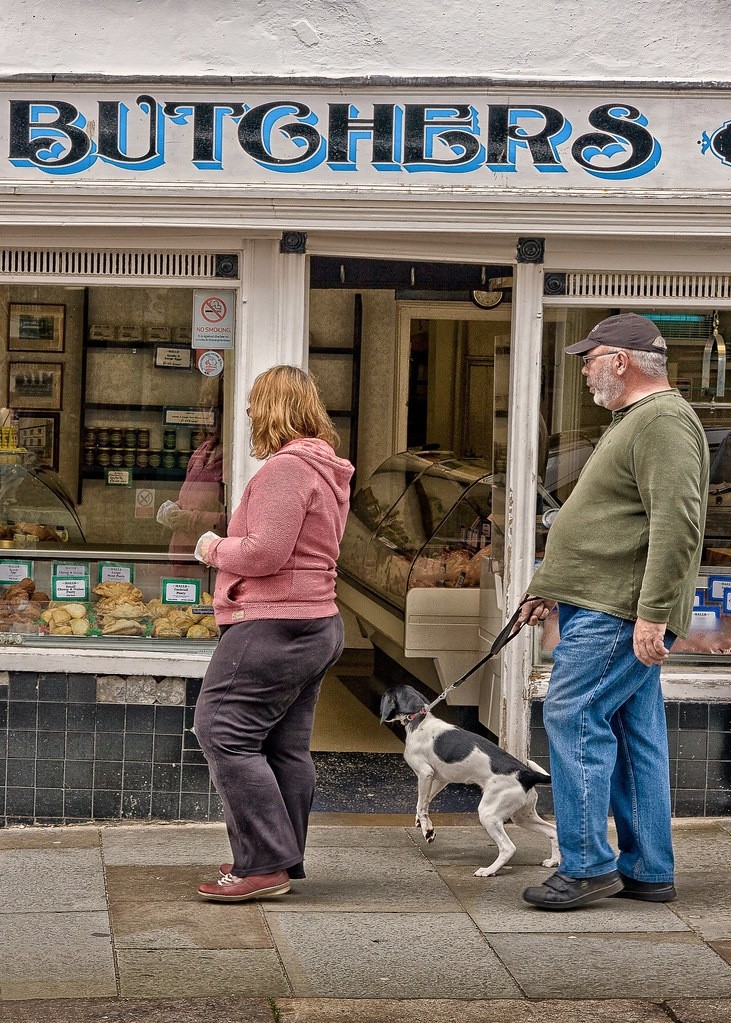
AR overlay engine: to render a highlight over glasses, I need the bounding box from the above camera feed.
[246,407,252,418]
[582,352,630,366]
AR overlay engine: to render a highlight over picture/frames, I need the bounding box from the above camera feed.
[11,413,61,472]
[7,303,66,352]
[8,361,64,410]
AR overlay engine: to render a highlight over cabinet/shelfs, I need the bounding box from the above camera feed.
[76,287,226,505]
[309,292,364,498]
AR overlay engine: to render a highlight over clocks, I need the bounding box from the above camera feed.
[471,291,504,310]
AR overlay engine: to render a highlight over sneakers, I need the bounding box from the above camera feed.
[219,863,235,876]
[197,867,291,902]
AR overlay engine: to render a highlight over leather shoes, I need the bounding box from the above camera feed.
[607,871,678,902]
[523,868,624,908]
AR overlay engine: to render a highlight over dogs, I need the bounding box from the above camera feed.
[377,686,562,878]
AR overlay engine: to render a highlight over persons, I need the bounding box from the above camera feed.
[168,373,226,596]
[513,313,710,909]
[0,446,83,542]
[194,365,355,901]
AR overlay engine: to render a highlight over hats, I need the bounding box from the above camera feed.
[563,312,667,355]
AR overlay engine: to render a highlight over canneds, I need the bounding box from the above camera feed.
[83,426,214,470]
